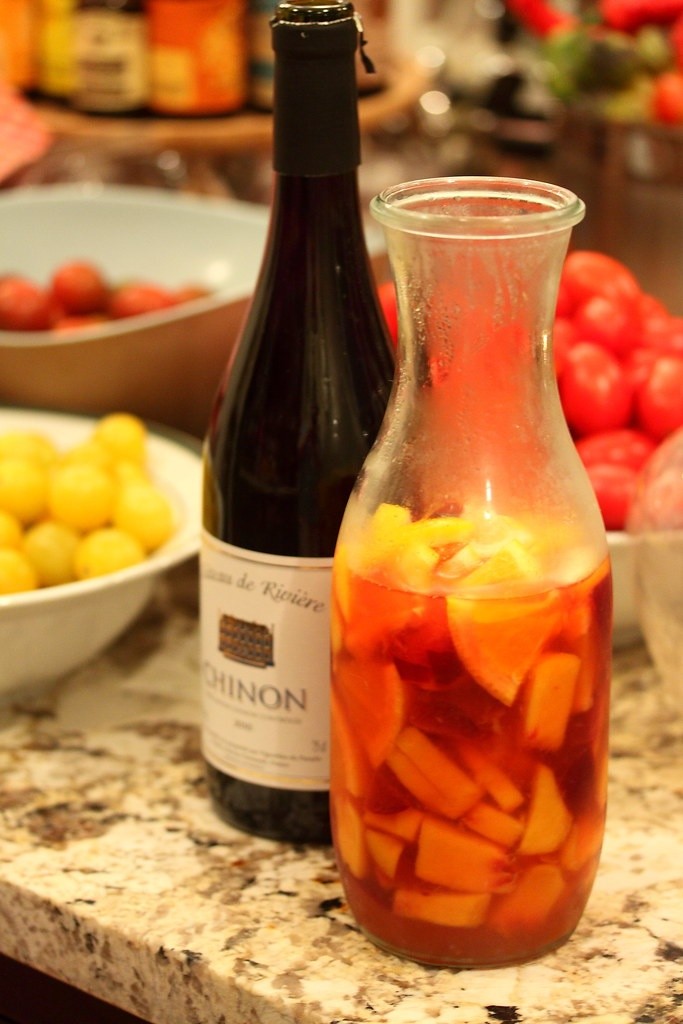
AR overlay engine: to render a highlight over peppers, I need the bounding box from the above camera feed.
[495,0,683,127]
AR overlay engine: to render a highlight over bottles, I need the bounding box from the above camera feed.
[324,172,618,970]
[199,0,422,846]
[27,0,386,120]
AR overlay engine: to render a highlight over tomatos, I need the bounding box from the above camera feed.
[0,413,176,596]
[377,252,683,531]
[1,261,210,336]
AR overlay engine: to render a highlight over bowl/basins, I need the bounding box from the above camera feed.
[1,178,291,435]
[605,526,683,717]
[1,403,226,700]
